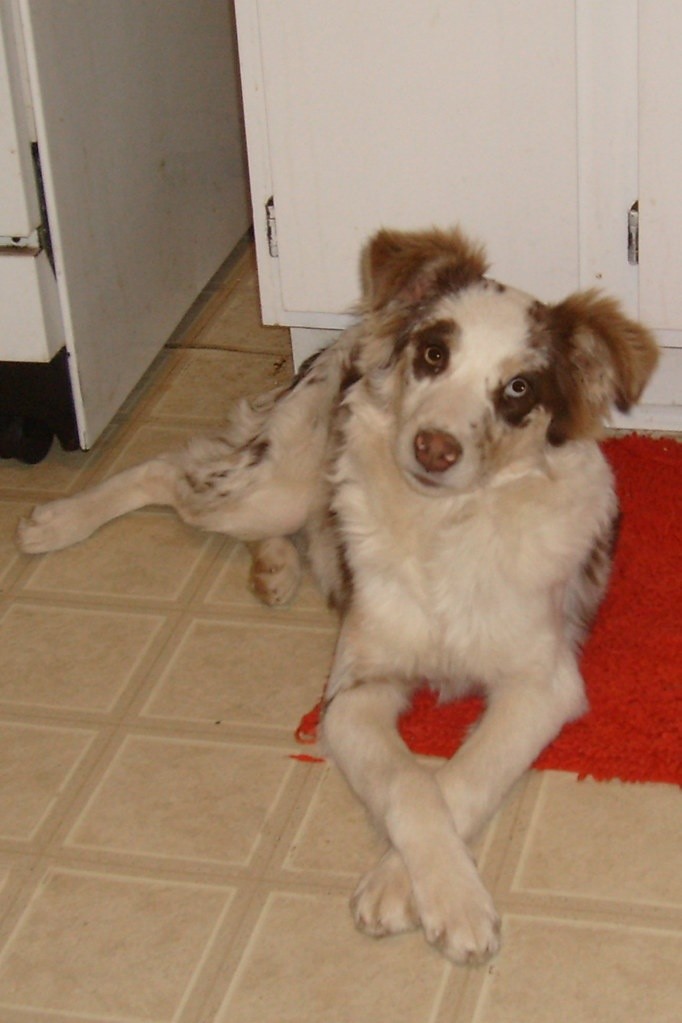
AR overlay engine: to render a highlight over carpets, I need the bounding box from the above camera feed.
[292,432,682,788]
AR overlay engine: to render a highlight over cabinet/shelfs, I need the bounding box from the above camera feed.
[0,0,248,452]
[235,0,682,432]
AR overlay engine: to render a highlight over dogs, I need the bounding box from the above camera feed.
[15,227,659,966]
